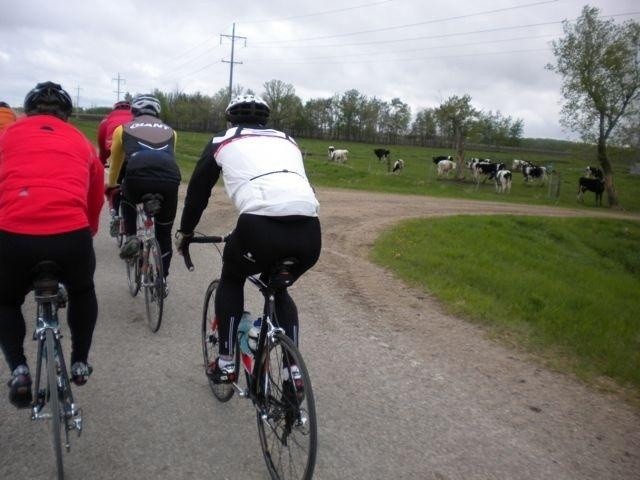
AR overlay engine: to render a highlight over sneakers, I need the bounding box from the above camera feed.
[155,277,170,298]
[119,235,140,259]
[109,216,120,237]
[278,379,307,404]
[71,361,93,385]
[8,373,33,408]
[204,358,238,383]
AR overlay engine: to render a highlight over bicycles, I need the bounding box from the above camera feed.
[102,162,141,268]
[14,256,88,479]
[182,231,319,480]
[111,191,172,337]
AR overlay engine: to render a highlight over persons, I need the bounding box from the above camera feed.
[0,80,106,409]
[0,100,17,132]
[105,93,183,296]
[177,92,324,402]
[97,99,134,237]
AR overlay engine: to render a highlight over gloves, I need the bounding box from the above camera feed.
[174,227,193,255]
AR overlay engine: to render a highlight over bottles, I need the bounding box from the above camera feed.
[248,317,265,352]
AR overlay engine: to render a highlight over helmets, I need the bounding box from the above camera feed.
[131,94,163,114]
[112,98,130,108]
[23,83,72,107]
[225,94,271,115]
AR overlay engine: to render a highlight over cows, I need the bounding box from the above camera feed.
[393,158,405,176]
[374,148,391,160]
[327,145,349,163]
[464,157,512,194]
[584,165,605,181]
[432,155,457,177]
[577,177,606,208]
[512,159,547,184]
